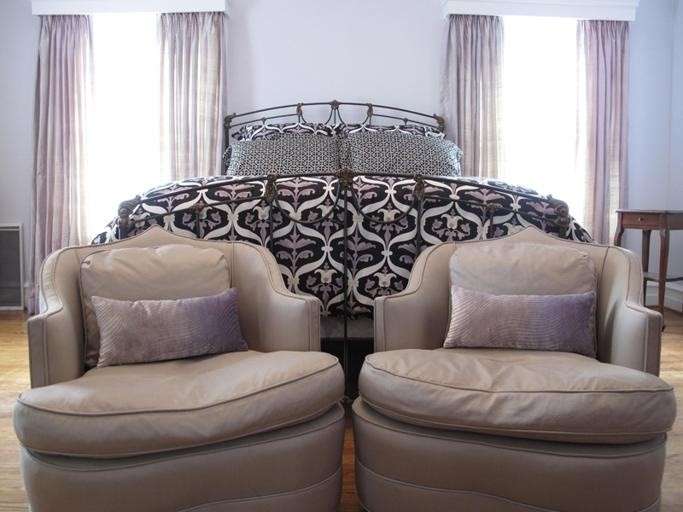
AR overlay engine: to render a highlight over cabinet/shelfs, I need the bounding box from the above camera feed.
[0,222,24,312]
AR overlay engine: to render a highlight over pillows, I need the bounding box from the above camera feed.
[231,122,339,142]
[77,243,232,368]
[89,285,248,370]
[222,135,340,176]
[444,239,598,356]
[335,123,446,139]
[441,282,600,356]
[341,131,464,177]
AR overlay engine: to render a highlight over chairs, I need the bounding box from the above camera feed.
[12,223,348,511]
[349,222,677,512]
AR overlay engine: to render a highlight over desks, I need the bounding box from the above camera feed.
[611,208,682,333]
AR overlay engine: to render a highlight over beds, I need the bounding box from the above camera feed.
[85,99,598,404]
